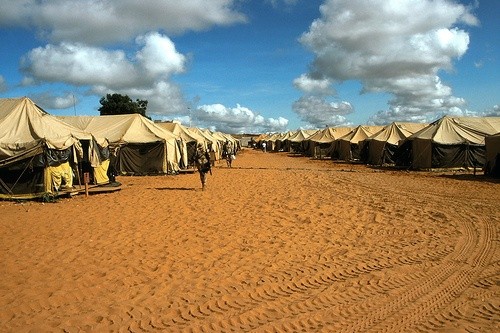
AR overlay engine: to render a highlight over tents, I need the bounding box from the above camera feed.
[0,95,499,199]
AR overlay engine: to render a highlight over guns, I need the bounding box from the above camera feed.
[205,149,212,175]
